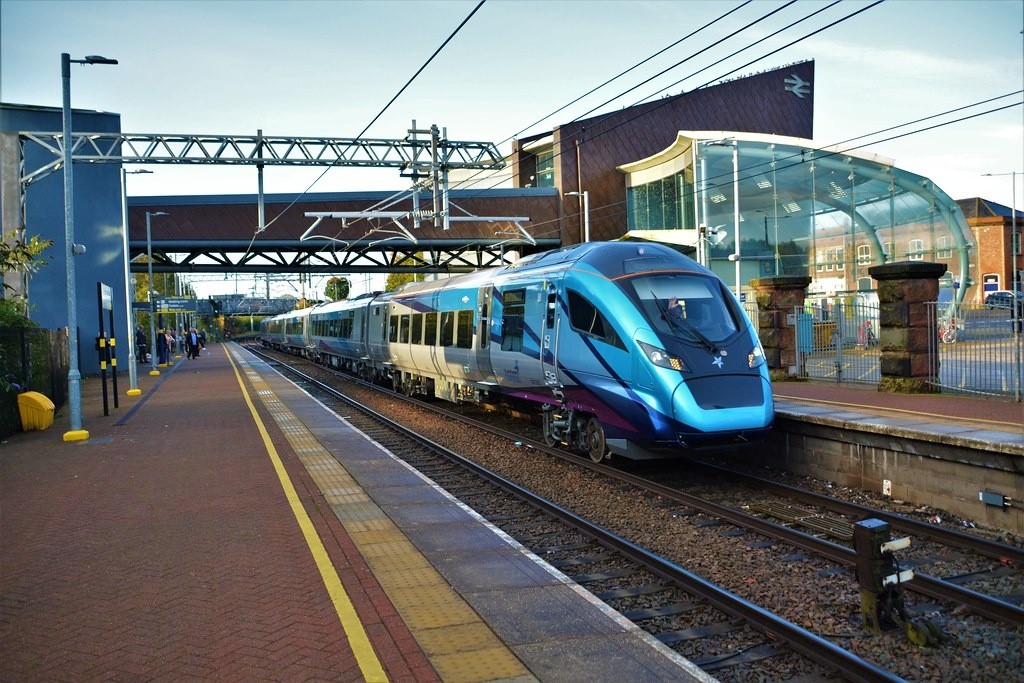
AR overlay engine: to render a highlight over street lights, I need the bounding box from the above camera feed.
[145,211,170,375]
[118,167,154,396]
[61,52,120,442]
[564,190,590,244]
[160,252,199,365]
[698,224,728,268]
[979,171,1024,403]
[707,139,741,304]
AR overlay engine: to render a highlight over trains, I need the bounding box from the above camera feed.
[260,240,773,464]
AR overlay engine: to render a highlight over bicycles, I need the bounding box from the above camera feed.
[864,316,876,349]
[937,316,959,344]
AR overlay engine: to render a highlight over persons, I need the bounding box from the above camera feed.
[666,296,682,326]
[136,325,150,363]
[157,327,206,363]
[99,331,111,378]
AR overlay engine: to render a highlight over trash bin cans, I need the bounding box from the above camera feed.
[17,391,56,431]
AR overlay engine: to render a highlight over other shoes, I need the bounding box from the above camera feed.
[187,356,190,360]
[193,358,196,359]
[144,361,151,363]
[140,362,145,363]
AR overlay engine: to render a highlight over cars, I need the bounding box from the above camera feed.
[983,290,1024,314]
